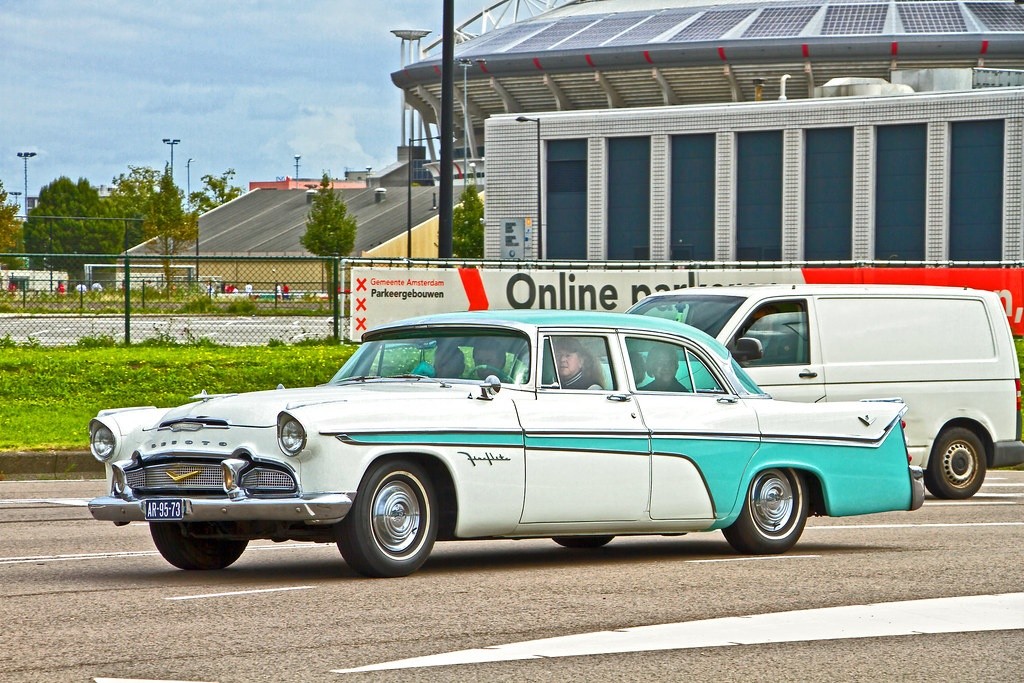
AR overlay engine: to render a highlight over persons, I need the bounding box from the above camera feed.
[553,337,605,390]
[741,305,798,365]
[76,281,87,292]
[276,282,282,293]
[628,350,646,390]
[473,339,506,380]
[433,344,465,378]
[91,281,102,292]
[8,280,18,291]
[243,282,253,292]
[118,278,239,293]
[281,281,289,294]
[637,342,690,393]
[57,282,66,293]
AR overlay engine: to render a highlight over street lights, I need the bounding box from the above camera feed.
[163,138,179,182]
[15,150,38,252]
[8,191,20,207]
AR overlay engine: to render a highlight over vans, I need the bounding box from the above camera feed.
[628,283,1021,499]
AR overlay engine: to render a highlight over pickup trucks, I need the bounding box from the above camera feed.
[83,305,924,573]
